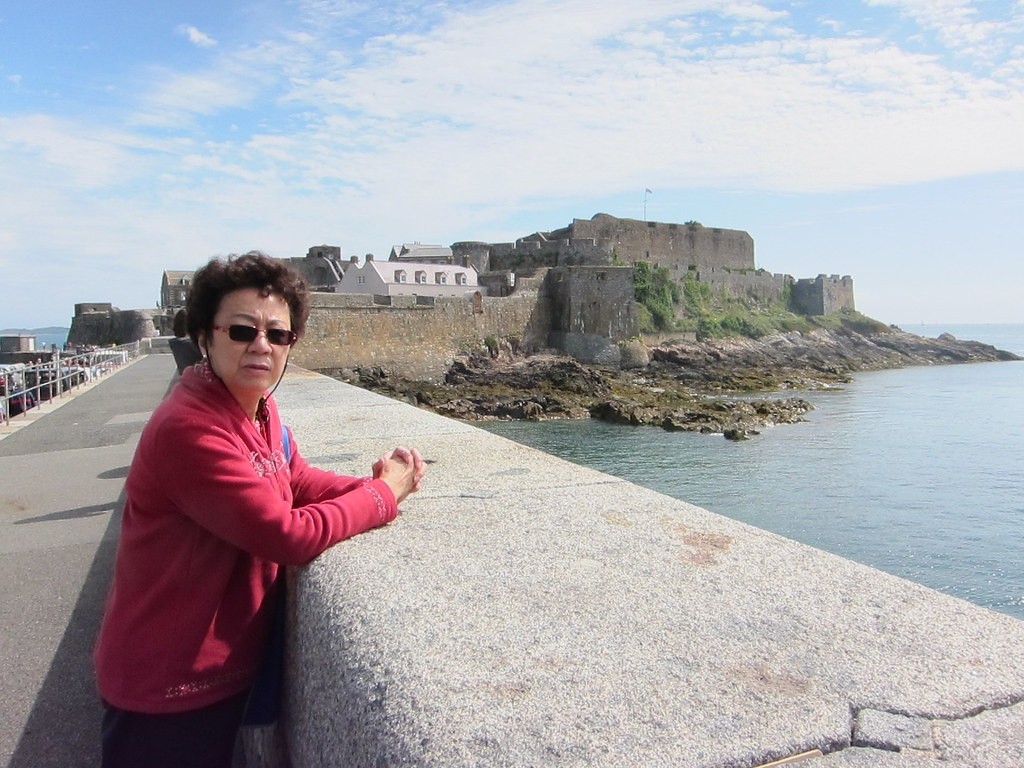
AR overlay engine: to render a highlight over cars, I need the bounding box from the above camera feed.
[0,367,35,419]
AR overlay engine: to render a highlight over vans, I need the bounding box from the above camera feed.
[22,367,89,401]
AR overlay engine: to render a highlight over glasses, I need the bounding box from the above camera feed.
[213,324,298,345]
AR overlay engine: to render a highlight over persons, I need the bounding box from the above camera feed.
[95,255,425,768]
